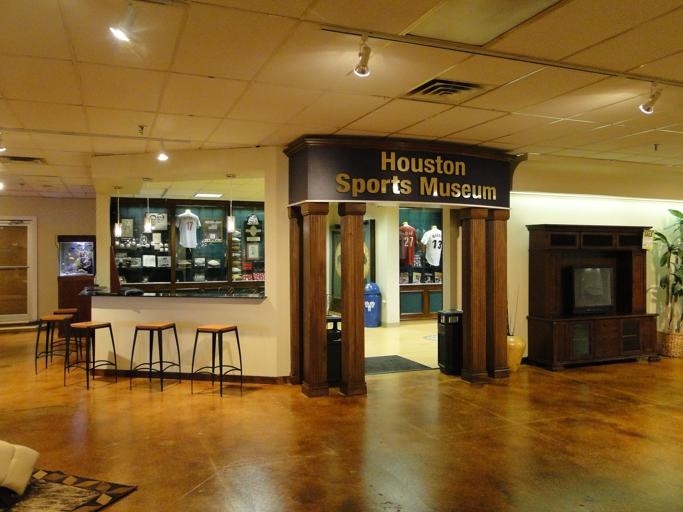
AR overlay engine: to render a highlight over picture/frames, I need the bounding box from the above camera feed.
[246,242,260,261]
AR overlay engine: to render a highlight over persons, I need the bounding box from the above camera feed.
[421,224,443,267]
[150,215,156,225]
[399,221,416,267]
[175,209,201,248]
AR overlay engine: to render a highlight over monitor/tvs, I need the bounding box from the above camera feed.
[562,265,616,316]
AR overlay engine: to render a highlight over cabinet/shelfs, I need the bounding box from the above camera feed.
[522,224,659,372]
[109,195,265,292]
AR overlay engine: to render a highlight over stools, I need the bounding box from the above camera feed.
[191,324,242,397]
[130,321,181,391]
[63,321,117,390]
[35,314,78,374]
[49,307,83,364]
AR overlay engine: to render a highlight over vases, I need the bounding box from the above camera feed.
[507,336,526,372]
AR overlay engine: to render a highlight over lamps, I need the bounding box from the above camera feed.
[354,43,370,75]
[639,91,661,113]
[108,3,138,41]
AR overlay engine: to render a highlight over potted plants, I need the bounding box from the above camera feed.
[652,208,683,357]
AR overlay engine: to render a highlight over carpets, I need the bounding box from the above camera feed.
[0,468,138,511]
[365,355,433,375]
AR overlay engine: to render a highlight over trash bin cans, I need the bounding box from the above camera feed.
[437,309,463,375]
[326,317,341,387]
[364,282,381,327]
